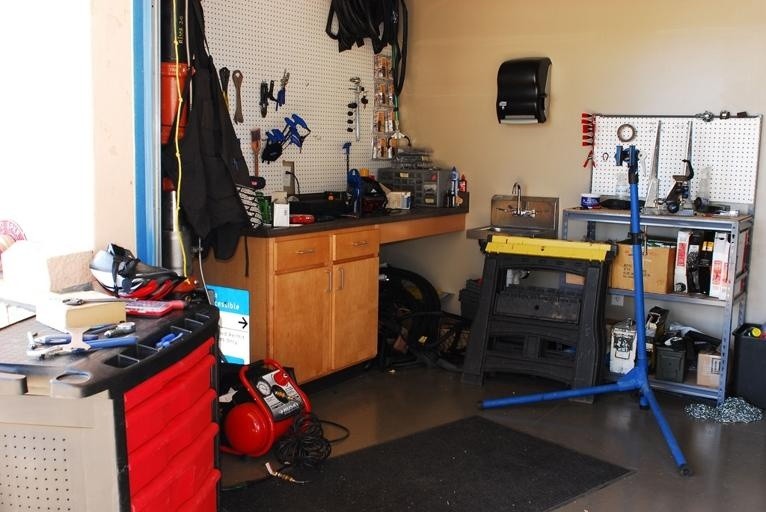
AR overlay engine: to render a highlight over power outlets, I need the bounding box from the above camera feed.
[283,168,291,187]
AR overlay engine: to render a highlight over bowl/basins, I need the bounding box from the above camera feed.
[580,194,601,208]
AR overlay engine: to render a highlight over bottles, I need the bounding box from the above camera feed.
[259,196,272,224]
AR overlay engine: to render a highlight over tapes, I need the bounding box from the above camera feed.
[617,124,634,142]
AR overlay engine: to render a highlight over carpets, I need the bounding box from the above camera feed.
[220,417,637,512]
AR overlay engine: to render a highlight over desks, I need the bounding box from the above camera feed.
[191,213,466,394]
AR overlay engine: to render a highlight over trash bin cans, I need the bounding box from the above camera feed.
[732,323,766,410]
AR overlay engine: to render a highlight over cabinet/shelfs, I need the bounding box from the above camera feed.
[561,206,754,409]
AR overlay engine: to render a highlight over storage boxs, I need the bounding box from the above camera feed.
[611,240,677,295]
[566,261,611,287]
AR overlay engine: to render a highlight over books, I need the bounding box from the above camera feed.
[35,291,126,334]
